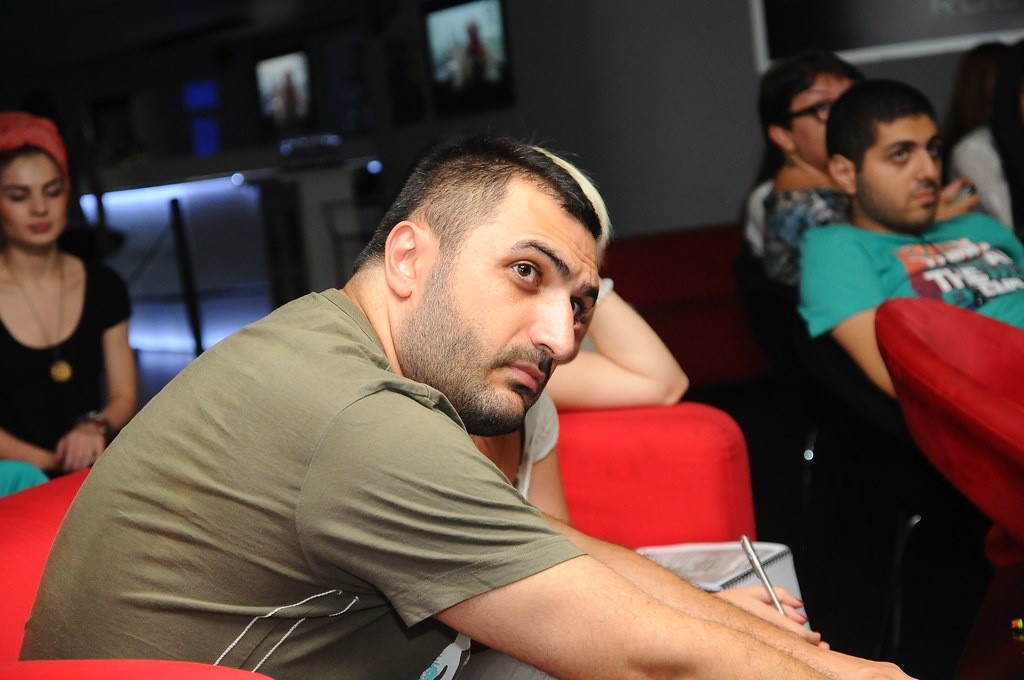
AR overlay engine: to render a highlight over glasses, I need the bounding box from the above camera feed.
[789,98,834,123]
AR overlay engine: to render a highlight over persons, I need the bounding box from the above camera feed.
[0,110,141,498]
[19,119,921,680]
[741,38,1024,400]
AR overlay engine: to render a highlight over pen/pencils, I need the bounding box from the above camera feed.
[739,535,788,620]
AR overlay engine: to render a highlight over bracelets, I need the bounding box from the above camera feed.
[595,277,613,303]
[84,410,116,444]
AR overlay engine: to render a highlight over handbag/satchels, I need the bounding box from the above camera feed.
[452,534,812,680]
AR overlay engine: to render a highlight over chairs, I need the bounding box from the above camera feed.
[873,296,1024,680]
[0,400,754,680]
[600,222,772,392]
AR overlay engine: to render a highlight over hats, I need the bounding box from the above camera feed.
[0,112,71,197]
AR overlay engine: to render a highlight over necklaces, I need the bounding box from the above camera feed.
[3,257,71,380]
